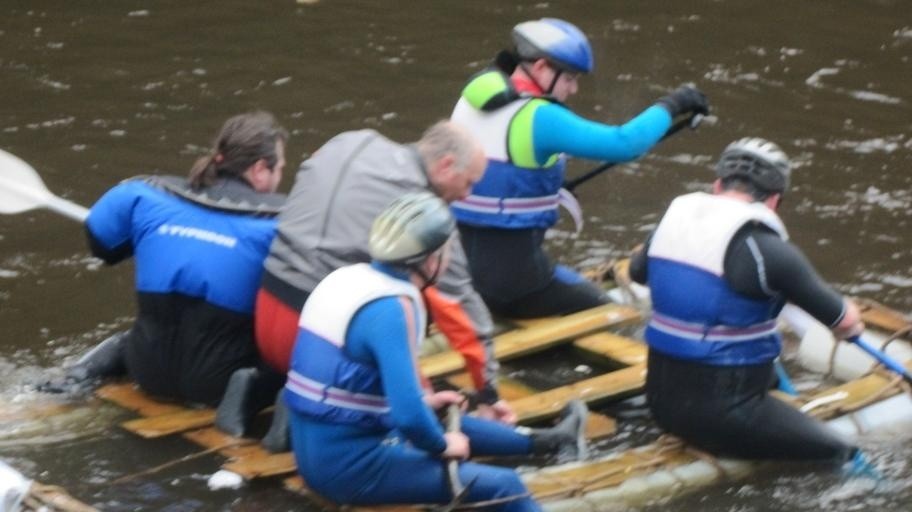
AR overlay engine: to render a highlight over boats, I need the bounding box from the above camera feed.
[33,257,911,512]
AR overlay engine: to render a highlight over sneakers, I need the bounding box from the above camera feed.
[544,397,587,462]
[261,386,291,452]
[215,365,261,437]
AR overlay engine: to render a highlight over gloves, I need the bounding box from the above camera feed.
[658,80,710,117]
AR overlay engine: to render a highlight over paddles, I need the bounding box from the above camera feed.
[431,377,478,512]
[0,147,89,222]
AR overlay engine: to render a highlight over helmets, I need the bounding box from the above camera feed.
[368,189,456,266]
[511,15,594,75]
[715,136,791,199]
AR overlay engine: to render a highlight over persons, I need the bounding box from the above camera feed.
[445,17,709,322]
[630,134,876,477]
[33,109,296,403]
[211,117,522,454]
[283,190,588,511]
